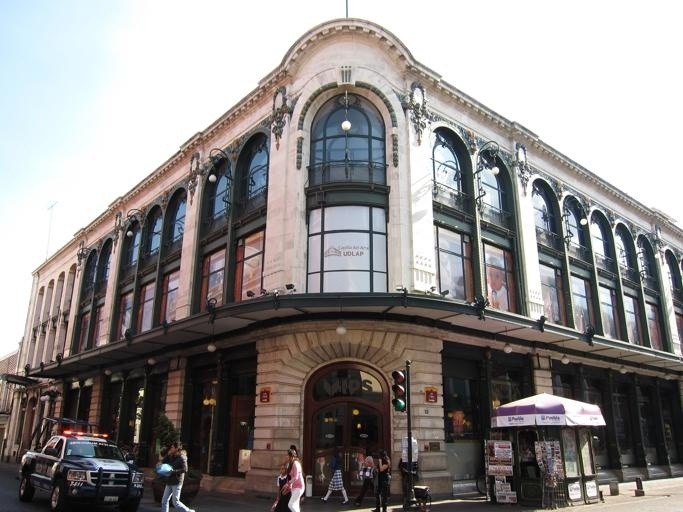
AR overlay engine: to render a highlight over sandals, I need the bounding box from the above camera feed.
[320,497,327,502]
[341,500,349,505]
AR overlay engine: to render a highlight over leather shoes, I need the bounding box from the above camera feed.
[354,503,361,506]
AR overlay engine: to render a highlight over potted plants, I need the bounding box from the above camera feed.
[151,411,204,507]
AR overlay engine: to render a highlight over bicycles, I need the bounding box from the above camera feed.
[476,468,487,496]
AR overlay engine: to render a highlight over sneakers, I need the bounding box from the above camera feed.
[371,508,387,512]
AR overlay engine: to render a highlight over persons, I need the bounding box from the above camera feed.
[319,446,350,505]
[371,448,392,512]
[355,447,364,481]
[285,444,298,469]
[353,455,378,507]
[270,464,292,512]
[280,448,305,512]
[317,450,326,479]
[154,439,195,512]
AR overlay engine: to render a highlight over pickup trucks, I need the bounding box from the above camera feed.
[19,430,144,512]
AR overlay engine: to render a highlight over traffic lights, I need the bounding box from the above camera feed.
[392,369,406,412]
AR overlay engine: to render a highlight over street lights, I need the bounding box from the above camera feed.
[203,398,217,473]
[40,381,63,441]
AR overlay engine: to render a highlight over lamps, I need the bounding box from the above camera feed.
[474,140,500,220]
[126,208,149,256]
[564,193,588,251]
[341,89,352,179]
[209,148,233,208]
